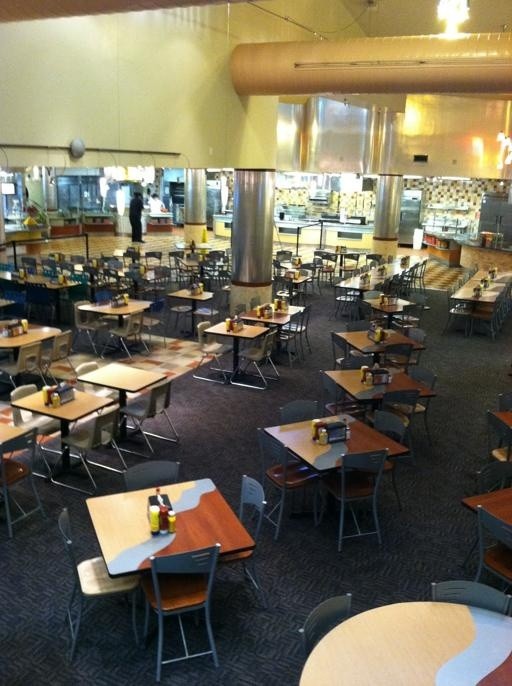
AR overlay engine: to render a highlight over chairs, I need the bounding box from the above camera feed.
[302,593,351,661]
[323,448,382,555]
[219,474,268,611]
[430,580,511,616]
[462,393,510,593]
[270,244,426,320]
[58,508,142,660]
[279,400,318,461]
[196,302,313,392]
[1,234,235,360]
[373,411,403,512]
[442,264,510,342]
[144,543,220,682]
[258,428,319,541]
[1,330,184,538]
[316,292,437,444]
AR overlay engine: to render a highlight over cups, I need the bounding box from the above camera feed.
[279,212,284,220]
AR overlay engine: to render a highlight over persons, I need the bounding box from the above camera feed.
[148,193,166,214]
[129,192,147,244]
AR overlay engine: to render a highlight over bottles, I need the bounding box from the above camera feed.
[473,267,498,297]
[360,365,392,384]
[311,416,351,443]
[192,283,204,295]
[44,386,61,406]
[287,257,302,280]
[111,293,130,308]
[226,298,289,333]
[370,292,388,343]
[4,317,28,337]
[359,256,409,284]
[149,505,176,535]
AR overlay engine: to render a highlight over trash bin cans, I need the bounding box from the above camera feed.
[479,231,505,250]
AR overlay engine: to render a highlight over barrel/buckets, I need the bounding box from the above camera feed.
[493,232,503,249]
[482,232,494,248]
